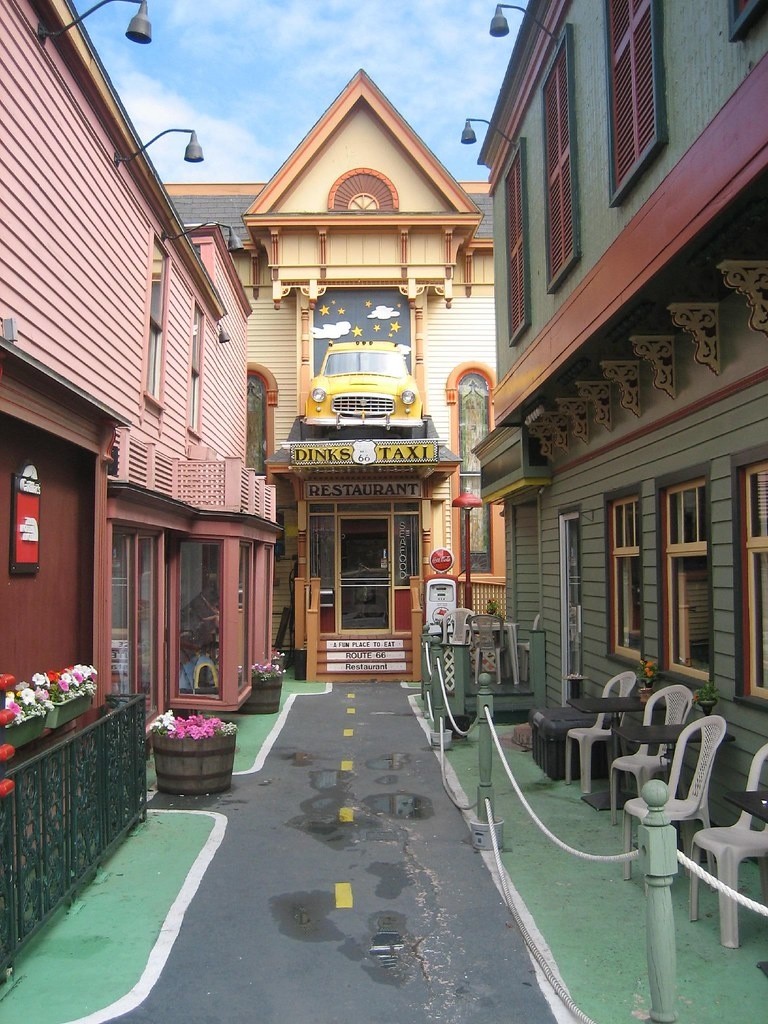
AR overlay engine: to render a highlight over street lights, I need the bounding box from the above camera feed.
[449,487,483,611]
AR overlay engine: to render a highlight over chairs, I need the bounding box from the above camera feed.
[623,715,726,892]
[442,607,476,646]
[517,614,541,681]
[468,614,508,685]
[689,743,768,948]
[566,671,636,793]
[611,684,695,824]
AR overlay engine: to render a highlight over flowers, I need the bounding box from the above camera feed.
[149,709,239,740]
[691,679,720,705]
[252,651,287,682]
[637,659,661,684]
[4,663,98,725]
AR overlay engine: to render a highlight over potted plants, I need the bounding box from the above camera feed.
[434,619,452,633]
[486,598,498,614]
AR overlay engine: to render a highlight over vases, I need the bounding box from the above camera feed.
[46,694,92,729]
[232,677,283,714]
[151,735,237,795]
[640,678,654,702]
[698,700,717,716]
[5,712,44,749]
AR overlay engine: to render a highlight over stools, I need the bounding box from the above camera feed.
[528,706,617,781]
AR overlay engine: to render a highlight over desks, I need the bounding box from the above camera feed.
[429,622,519,685]
[721,789,768,825]
[565,696,667,810]
[611,724,735,854]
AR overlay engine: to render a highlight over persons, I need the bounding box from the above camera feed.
[186,573,220,656]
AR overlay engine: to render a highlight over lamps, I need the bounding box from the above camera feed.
[39,0,152,44]
[161,221,244,252]
[217,323,231,343]
[490,5,559,46]
[114,129,204,168]
[461,119,521,149]
